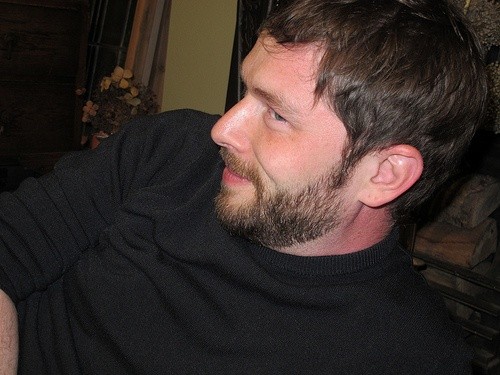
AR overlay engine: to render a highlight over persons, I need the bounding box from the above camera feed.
[0,0,489,375]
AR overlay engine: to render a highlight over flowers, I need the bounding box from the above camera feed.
[82,66,158,146]
